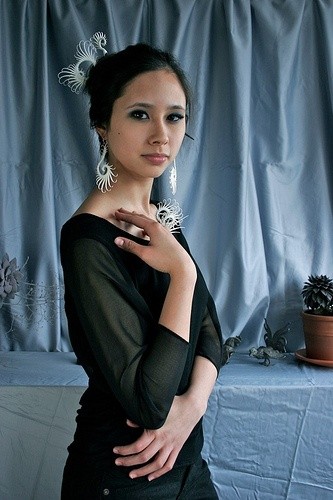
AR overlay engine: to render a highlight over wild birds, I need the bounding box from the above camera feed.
[223,336,288,367]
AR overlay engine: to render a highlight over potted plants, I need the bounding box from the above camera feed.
[301,273,333,360]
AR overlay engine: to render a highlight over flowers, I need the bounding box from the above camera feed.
[0,252,24,303]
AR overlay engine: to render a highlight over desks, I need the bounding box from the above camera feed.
[0,351,333,500]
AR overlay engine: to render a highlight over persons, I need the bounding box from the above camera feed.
[57,39,228,500]
